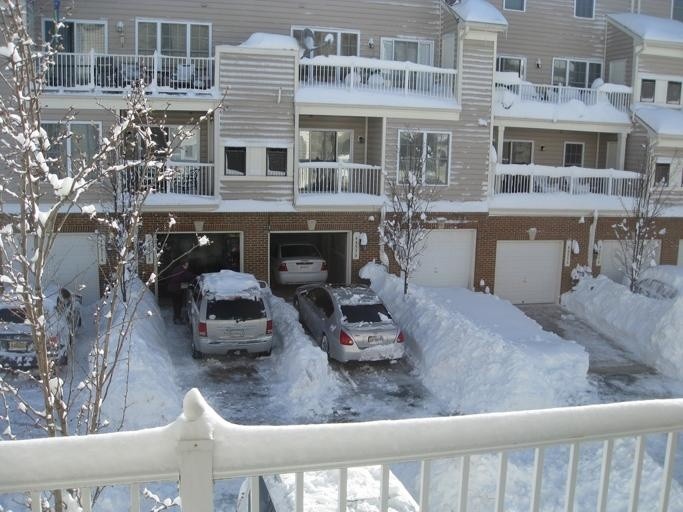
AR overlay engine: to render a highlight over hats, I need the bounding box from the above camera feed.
[182,270,273,358]
[272,243,328,285]
[293,282,403,362]
[631,264,683,302]
[1,285,83,373]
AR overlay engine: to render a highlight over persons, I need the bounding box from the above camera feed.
[167,259,196,325]
